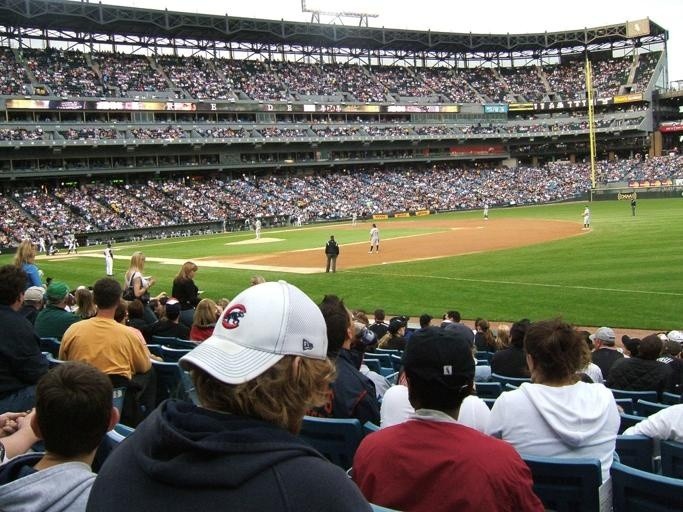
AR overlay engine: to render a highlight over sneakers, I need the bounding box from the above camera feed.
[324,271,336,273]
[368,251,379,254]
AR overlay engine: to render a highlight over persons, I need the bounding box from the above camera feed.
[315,296,682,511]
[253,217,262,240]
[0,47,372,512]
[324,236,340,273]
[250,56,682,229]
[367,224,380,254]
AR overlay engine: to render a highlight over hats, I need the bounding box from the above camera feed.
[402,326,475,381]
[351,323,377,345]
[23,281,69,301]
[388,317,407,333]
[589,327,616,344]
[657,330,683,342]
[177,278,330,385]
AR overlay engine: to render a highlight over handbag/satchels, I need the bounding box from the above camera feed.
[123,269,150,302]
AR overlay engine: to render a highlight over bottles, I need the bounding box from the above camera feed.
[354,322,377,345]
